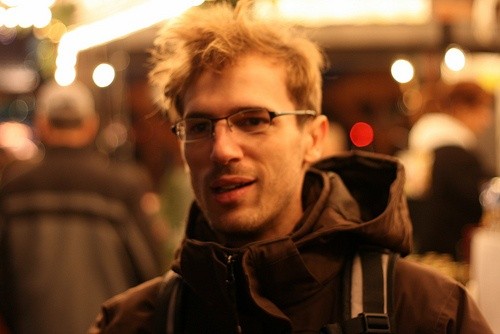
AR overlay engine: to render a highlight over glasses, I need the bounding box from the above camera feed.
[172,108,316,144]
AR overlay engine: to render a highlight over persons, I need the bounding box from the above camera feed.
[89,1,496,334]
[1,47,192,255]
[400,76,499,289]
